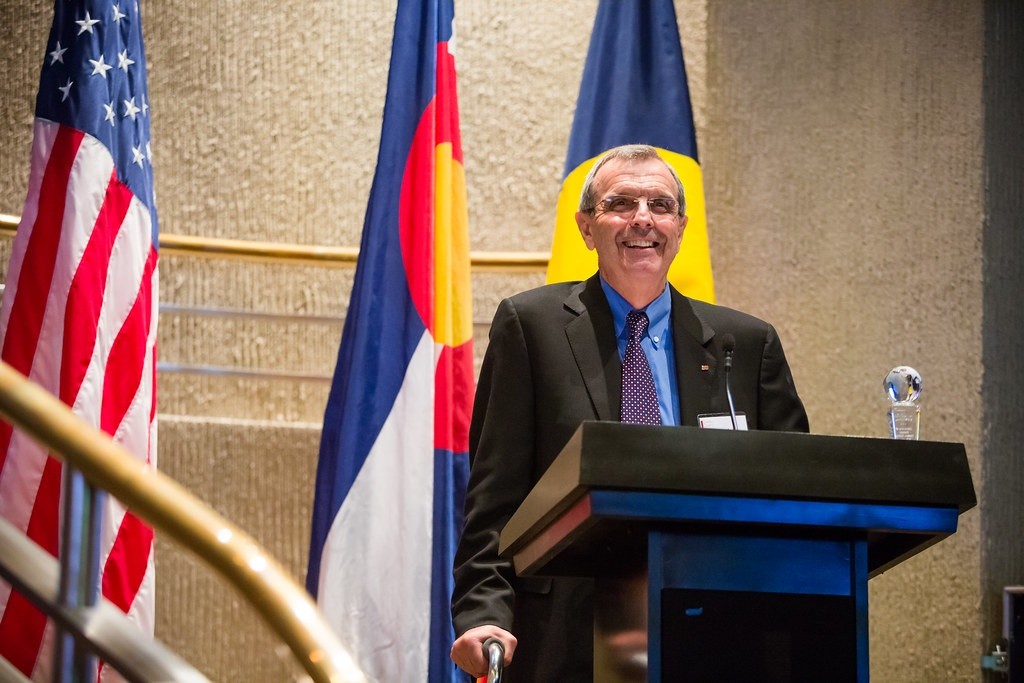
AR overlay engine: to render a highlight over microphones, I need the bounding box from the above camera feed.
[722,333,738,430]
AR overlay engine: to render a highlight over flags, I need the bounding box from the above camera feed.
[545,1,720,307]
[0,0,156,683]
[305,0,473,683]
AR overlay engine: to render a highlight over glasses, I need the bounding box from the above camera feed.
[588,195,681,216]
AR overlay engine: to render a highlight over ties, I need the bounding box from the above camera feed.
[619,310,661,426]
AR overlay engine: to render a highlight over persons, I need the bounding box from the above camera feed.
[449,144,810,675]
